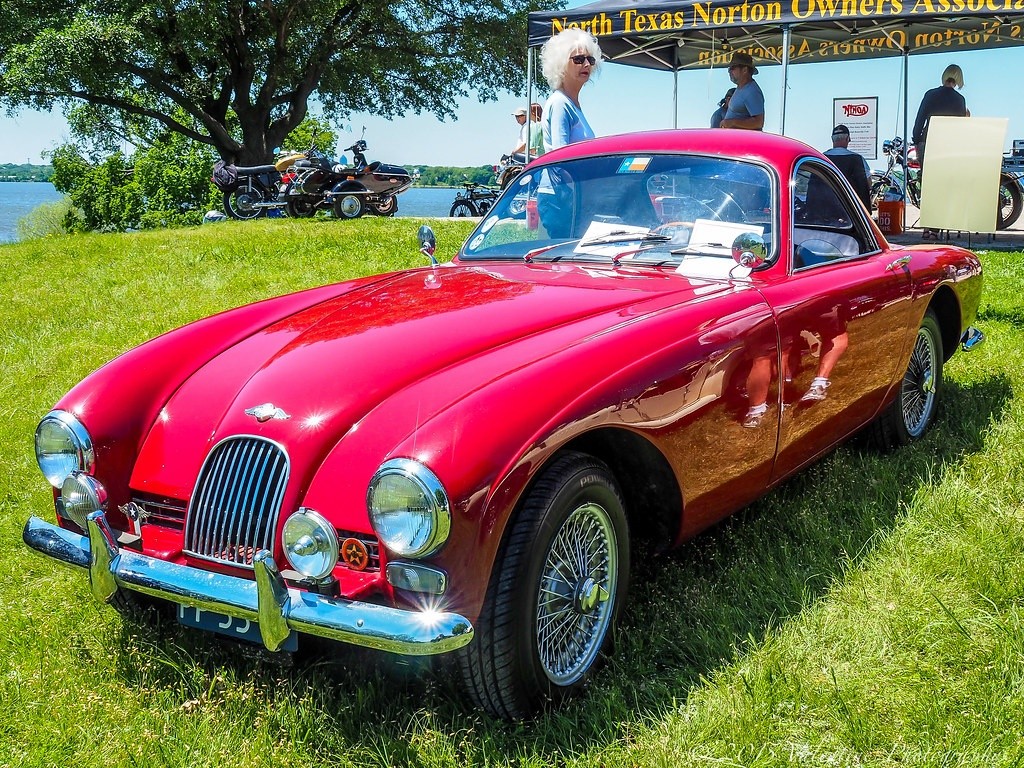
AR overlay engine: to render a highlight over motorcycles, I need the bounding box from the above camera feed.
[449,152,540,218]
[867,136,1024,231]
[209,124,421,218]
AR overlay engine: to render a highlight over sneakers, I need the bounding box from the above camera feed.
[800,381,832,402]
[743,405,770,428]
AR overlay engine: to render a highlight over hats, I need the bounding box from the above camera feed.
[729,53,759,75]
[511,109,527,115]
[833,125,851,142]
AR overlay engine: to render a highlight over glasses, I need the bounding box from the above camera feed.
[569,55,596,65]
[730,66,744,70]
[516,115,526,119]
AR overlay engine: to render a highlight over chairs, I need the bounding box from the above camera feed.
[805,154,872,226]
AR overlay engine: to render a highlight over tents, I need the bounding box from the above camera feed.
[528,0,1024,273]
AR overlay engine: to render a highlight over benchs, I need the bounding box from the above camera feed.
[762,227,860,260]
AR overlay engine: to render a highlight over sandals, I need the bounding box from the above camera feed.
[923,231,938,241]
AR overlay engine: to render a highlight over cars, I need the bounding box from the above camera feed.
[20,128,990,724]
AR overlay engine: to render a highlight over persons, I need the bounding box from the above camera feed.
[536,27,598,240]
[510,102,542,195]
[710,88,735,128]
[743,316,849,427]
[823,125,872,191]
[719,52,765,130]
[912,64,966,240]
